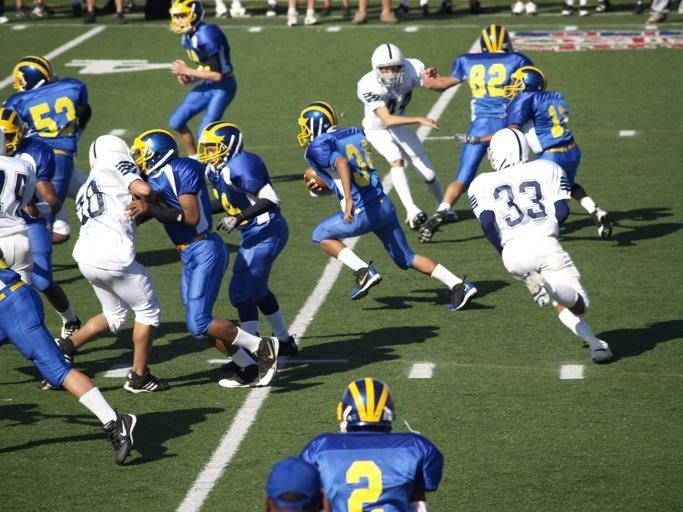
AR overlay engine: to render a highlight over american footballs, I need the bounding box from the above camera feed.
[304,168,333,194]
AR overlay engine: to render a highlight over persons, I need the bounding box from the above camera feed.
[296,373,446,512]
[461,126,618,367]
[126,129,279,388]
[167,0,238,163]
[451,62,615,242]
[0,55,92,339]
[262,455,326,512]
[1,1,683,28]
[0,247,137,465]
[40,135,171,394]
[198,121,298,363]
[298,98,479,312]
[415,22,535,244]
[354,42,461,231]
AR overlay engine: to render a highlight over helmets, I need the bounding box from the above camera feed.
[371,43,403,88]
[169,0,205,34]
[296,101,336,149]
[0,109,22,156]
[502,65,546,99]
[486,127,528,172]
[337,377,396,431]
[12,56,57,93]
[479,23,511,54]
[88,121,243,169]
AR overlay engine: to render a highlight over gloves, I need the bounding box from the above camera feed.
[454,133,480,145]
[216,213,243,233]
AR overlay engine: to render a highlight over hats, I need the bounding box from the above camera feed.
[266,457,321,509]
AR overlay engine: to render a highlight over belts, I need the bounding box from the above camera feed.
[0,280,25,301]
[544,140,576,153]
[175,228,213,251]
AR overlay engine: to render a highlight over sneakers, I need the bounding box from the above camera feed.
[406,205,457,243]
[590,338,613,362]
[349,260,382,301]
[123,369,168,394]
[39,338,73,390]
[103,414,137,464]
[217,335,297,390]
[447,275,478,312]
[595,210,611,239]
[61,315,80,339]
[522,271,552,307]
